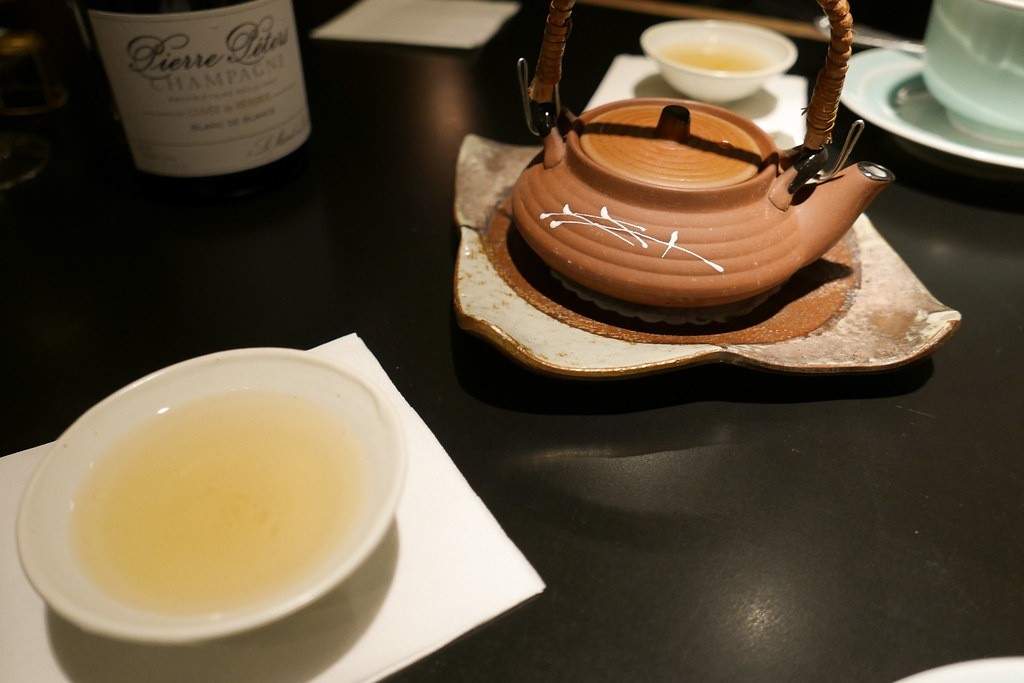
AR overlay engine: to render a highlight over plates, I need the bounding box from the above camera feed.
[839,44,1024,171]
[582,52,810,151]
[452,132,962,379]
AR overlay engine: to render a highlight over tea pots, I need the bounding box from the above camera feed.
[510,0,897,309]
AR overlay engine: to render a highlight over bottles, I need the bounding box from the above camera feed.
[75,0,324,221]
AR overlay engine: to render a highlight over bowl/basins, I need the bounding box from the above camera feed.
[16,345,401,645]
[921,1,1024,150]
[638,18,799,107]
